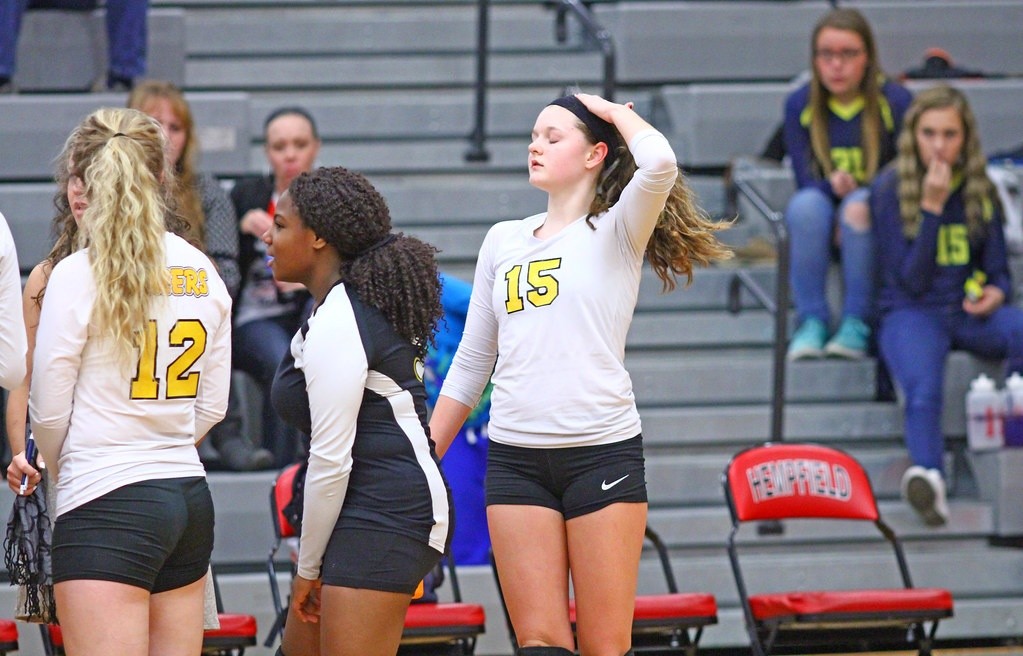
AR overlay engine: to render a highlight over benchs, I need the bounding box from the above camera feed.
[584,0,1023,493]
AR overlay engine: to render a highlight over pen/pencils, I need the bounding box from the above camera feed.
[19,433,38,496]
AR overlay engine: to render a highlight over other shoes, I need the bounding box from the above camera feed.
[900,464,951,526]
[785,315,827,362]
[823,316,873,361]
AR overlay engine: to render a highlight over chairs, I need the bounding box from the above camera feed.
[268,458,485,656]
[720,439,955,656]
[487,522,719,656]
[0,0,494,656]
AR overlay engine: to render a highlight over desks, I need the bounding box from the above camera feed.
[15,431,257,656]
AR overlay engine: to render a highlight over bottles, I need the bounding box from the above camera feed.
[1001,372,1023,450]
[966,372,1006,451]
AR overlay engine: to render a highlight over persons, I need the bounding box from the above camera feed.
[432,91,741,656]
[787,10,1023,527]
[0,0,499,656]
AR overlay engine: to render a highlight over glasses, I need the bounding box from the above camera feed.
[816,47,868,61]
